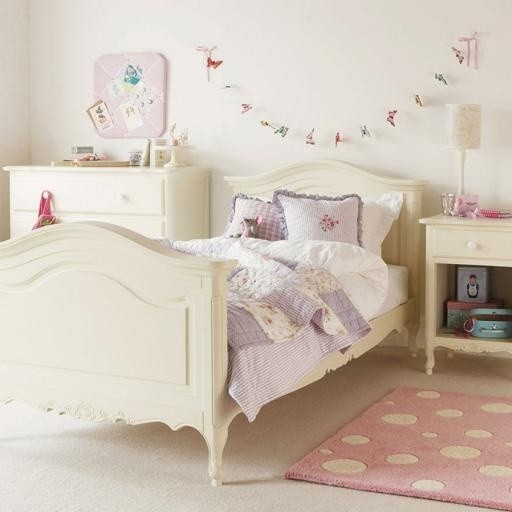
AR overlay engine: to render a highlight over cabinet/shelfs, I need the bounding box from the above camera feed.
[1,163,212,245]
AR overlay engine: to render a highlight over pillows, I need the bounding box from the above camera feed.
[272,188,364,247]
[224,192,286,243]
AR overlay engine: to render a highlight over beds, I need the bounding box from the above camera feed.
[0,156,432,485]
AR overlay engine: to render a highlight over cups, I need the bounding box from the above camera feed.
[438,192,456,216]
[128,149,144,166]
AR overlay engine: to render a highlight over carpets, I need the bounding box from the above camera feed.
[287,385,511,511]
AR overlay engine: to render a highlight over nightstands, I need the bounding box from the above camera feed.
[417,211,512,376]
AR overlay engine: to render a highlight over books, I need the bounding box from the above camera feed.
[481,210,512,218]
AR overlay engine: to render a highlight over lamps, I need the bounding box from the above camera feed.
[446,103,483,217]
